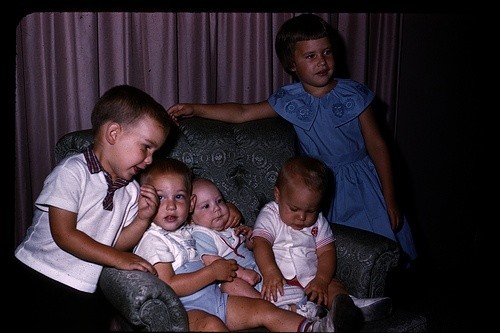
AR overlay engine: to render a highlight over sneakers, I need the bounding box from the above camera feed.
[312,293,364,333]
[296,301,328,321]
[351,297,394,322]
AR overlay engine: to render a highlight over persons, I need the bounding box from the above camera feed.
[12,84,172,331]
[166,14,418,271]
[131,156,366,331]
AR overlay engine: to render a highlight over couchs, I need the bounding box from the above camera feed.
[56,116,428,333]
